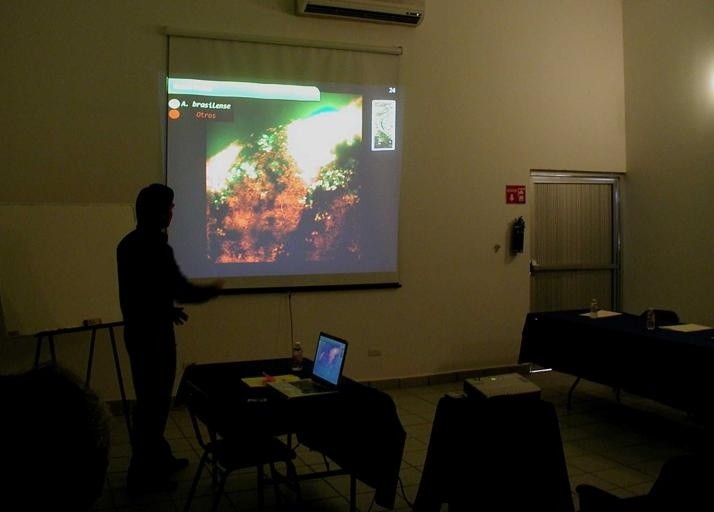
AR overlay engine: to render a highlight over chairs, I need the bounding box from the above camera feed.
[183,360,298,510]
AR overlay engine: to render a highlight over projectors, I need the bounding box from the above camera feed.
[463,372,541,405]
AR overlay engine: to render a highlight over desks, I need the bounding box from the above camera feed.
[519,312,710,429]
[180,357,406,509]
[416,392,575,510]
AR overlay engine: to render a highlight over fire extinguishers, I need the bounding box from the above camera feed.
[511,216,525,252]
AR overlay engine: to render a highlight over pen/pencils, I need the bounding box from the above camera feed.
[248,399,267,402]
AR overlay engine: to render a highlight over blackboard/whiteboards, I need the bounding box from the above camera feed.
[0,202,137,339]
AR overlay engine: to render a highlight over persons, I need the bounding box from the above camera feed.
[1,363,111,512]
[117,184,225,494]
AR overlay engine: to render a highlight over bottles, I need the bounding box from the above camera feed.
[590,299,598,320]
[645,308,656,331]
[290,341,304,372]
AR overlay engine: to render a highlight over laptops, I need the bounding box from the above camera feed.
[266,331,348,399]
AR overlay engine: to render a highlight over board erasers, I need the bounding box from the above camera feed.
[84,318,102,326]
